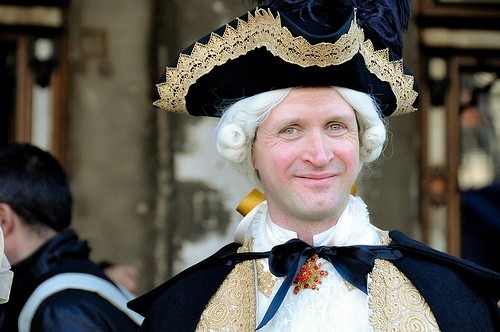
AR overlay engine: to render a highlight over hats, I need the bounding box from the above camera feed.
[151,0,421,119]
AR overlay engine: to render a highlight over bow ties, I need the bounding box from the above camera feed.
[222,239,403,331]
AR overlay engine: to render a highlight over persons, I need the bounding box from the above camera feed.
[123,0,500,331]
[0,142,147,332]
